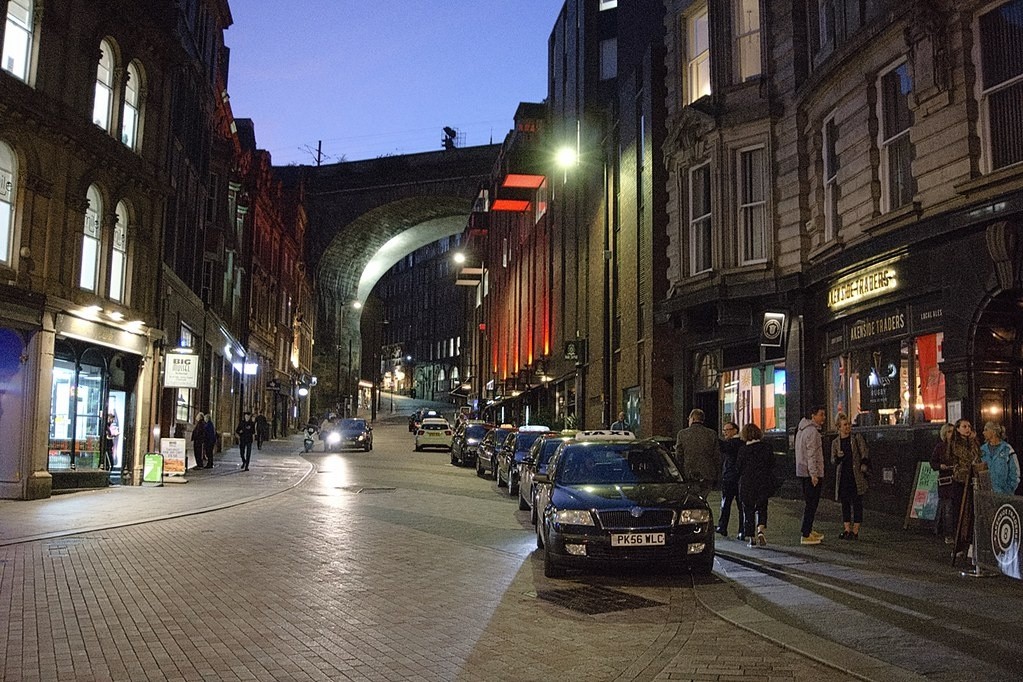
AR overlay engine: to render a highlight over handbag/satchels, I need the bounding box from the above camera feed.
[938,475,953,486]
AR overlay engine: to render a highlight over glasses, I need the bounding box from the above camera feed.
[723,427,734,431]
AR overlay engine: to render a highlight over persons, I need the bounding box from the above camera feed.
[894,410,903,424]
[308,412,343,453]
[675,409,775,549]
[611,412,630,431]
[105,414,120,471]
[795,405,826,545]
[235,411,267,471]
[930,418,1021,558]
[831,411,869,540]
[191,412,216,470]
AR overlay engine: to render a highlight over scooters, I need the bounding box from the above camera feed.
[301,421,321,453]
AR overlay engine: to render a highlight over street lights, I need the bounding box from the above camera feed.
[452,252,485,419]
[554,146,611,432]
[353,302,377,420]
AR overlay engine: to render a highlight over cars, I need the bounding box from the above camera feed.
[408,406,551,497]
[532,429,715,577]
[519,429,583,526]
[327,418,373,452]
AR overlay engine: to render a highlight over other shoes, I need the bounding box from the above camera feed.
[193,466,203,470]
[944,536,955,545]
[241,460,250,471]
[204,464,214,469]
[810,529,824,540]
[747,541,757,548]
[736,532,745,541]
[800,533,822,545]
[758,531,767,546]
[839,531,858,540]
[715,526,727,536]
